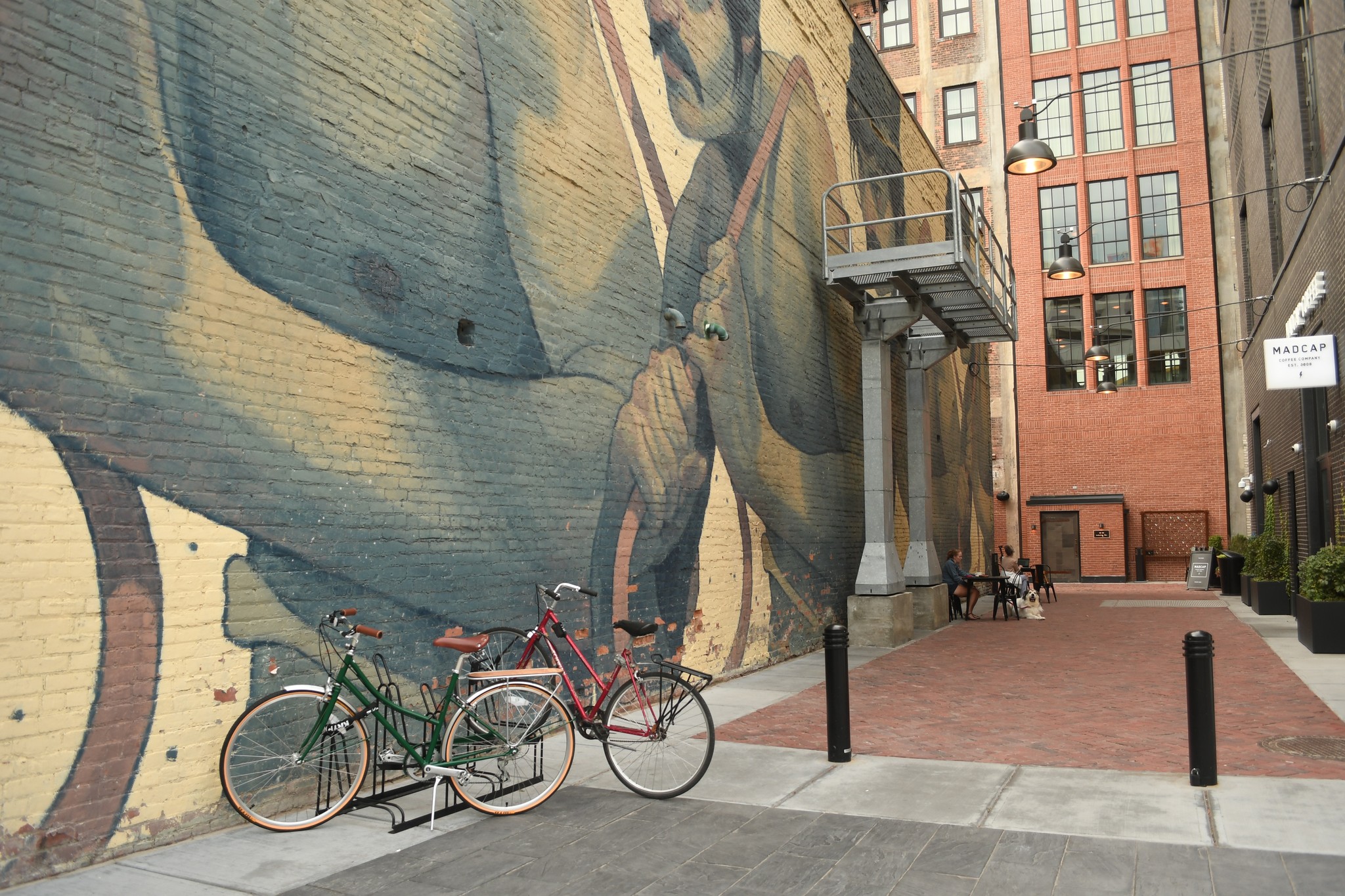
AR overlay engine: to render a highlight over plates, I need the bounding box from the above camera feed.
[975,576,979,577]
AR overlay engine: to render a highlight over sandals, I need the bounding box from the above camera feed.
[963,612,980,620]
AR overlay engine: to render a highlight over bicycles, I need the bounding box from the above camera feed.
[454,581,715,799]
[219,608,578,832]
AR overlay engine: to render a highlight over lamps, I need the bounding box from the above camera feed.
[1003,24,1345,176]
[1085,295,1274,359]
[1047,176,1331,281]
[1096,337,1254,393]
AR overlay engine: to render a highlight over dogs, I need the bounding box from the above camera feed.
[1002,588,1046,621]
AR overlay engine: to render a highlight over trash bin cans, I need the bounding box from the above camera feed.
[1216,550,1245,595]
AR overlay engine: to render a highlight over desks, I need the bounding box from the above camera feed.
[961,576,1010,621]
[1021,567,1036,583]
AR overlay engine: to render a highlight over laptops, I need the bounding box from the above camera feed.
[1017,558,1030,567]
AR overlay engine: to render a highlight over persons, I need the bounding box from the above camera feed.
[1000,544,1028,598]
[942,549,981,620]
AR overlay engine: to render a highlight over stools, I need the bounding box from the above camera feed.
[949,595,963,623]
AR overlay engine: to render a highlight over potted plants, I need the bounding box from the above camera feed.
[1240,536,1290,615]
[1216,554,1245,595]
[1296,544,1345,653]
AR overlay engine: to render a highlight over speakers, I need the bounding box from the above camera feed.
[996,492,1009,501]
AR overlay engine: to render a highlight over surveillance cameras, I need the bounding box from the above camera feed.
[1238,481,1246,489]
[1291,444,1299,453]
[1326,420,1336,433]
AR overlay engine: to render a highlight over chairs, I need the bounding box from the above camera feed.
[993,570,1020,621]
[995,563,1020,598]
[1030,564,1057,604]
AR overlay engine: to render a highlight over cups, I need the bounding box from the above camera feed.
[975,572,982,576]
